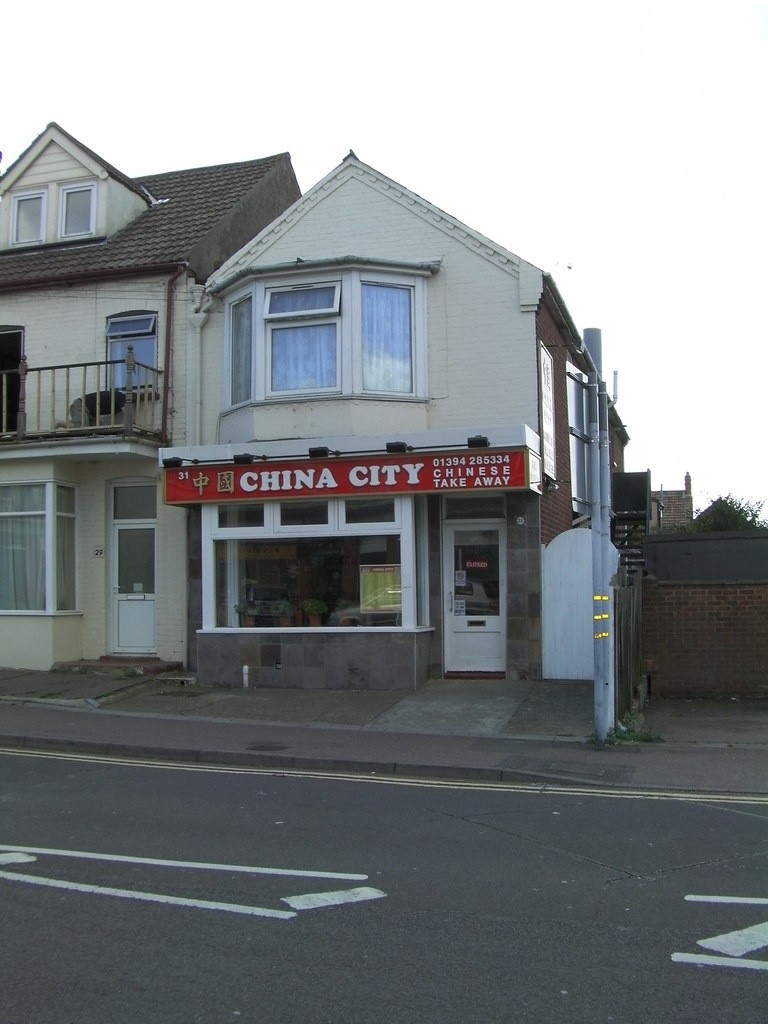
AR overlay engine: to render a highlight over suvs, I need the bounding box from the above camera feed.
[455,576,499,615]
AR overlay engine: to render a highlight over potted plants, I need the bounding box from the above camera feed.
[335,598,357,625]
[271,601,293,627]
[235,604,261,627]
[301,599,327,626]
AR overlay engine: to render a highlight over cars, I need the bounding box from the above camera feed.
[328,585,401,627]
[245,584,296,617]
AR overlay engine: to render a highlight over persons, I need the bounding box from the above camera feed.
[54,390,141,428]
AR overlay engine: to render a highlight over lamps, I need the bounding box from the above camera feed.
[467,435,490,448]
[162,456,182,467]
[386,442,408,453]
[233,454,253,464]
[308,447,329,457]
